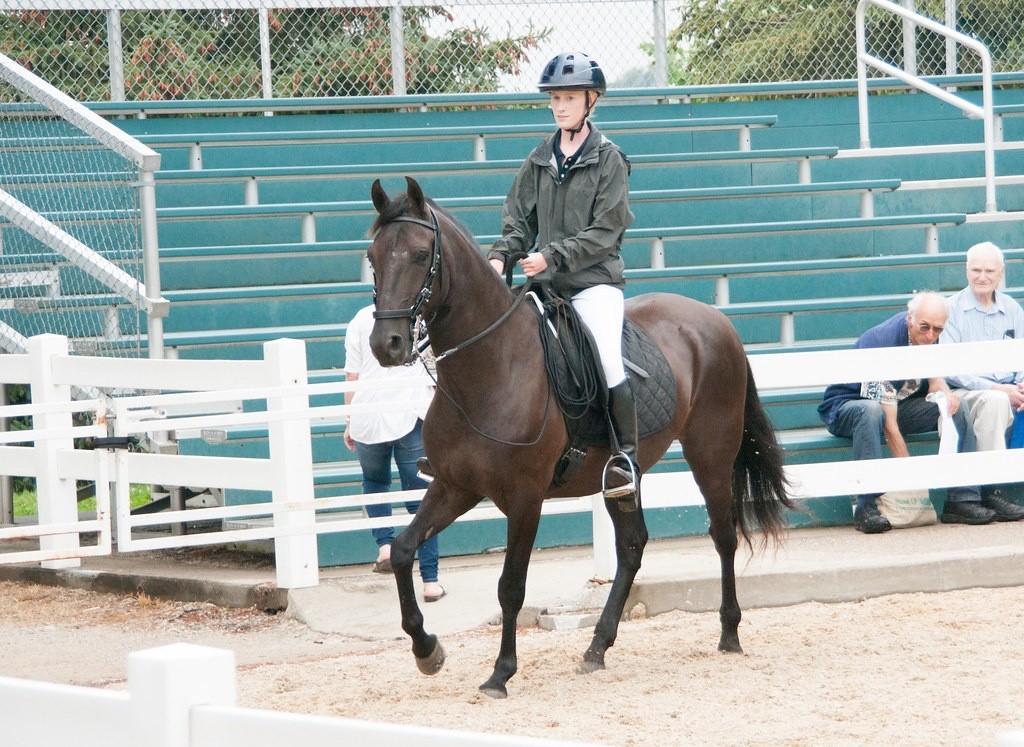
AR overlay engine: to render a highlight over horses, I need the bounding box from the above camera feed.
[364,173,812,698]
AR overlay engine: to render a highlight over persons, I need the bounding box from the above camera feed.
[486,50,641,513]
[818,290,996,533]
[344,304,448,602]
[938,242,1024,521]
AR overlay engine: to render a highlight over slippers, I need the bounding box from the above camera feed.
[372,556,395,575]
[423,584,449,603]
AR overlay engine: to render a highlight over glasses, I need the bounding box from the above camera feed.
[910,315,944,334]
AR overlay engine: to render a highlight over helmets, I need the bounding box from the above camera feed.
[536,50,608,142]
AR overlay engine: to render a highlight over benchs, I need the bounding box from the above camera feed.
[0,70,1024,566]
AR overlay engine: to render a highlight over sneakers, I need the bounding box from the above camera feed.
[852,500,892,534]
[940,489,1024,525]
[601,454,643,513]
